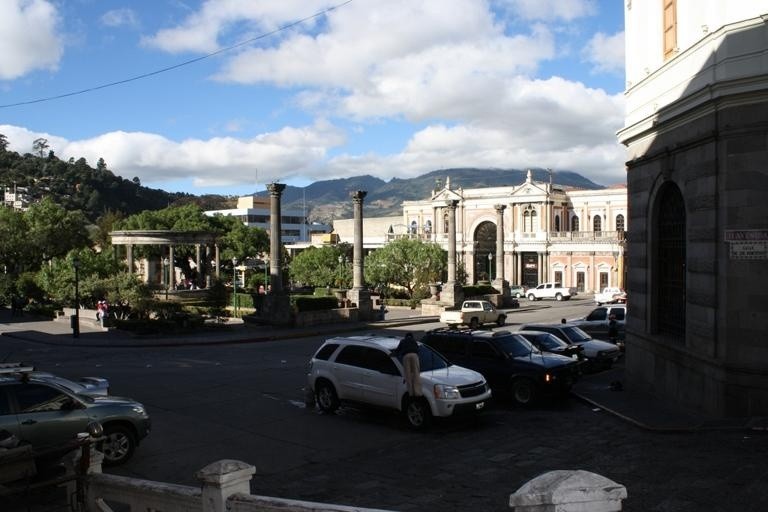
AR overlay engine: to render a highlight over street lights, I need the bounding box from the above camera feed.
[164,258,169,300]
[488,253,492,282]
[73,257,81,338]
[339,256,342,290]
[232,256,237,318]
[264,255,268,290]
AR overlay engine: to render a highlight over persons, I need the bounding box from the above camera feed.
[259,285,272,294]
[96,300,109,321]
[402,332,425,398]
[607,313,619,342]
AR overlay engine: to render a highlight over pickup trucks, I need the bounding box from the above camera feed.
[526,282,578,301]
[593,287,625,306]
[440,300,507,330]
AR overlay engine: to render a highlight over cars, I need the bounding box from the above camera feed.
[511,285,528,298]
[0,362,151,497]
